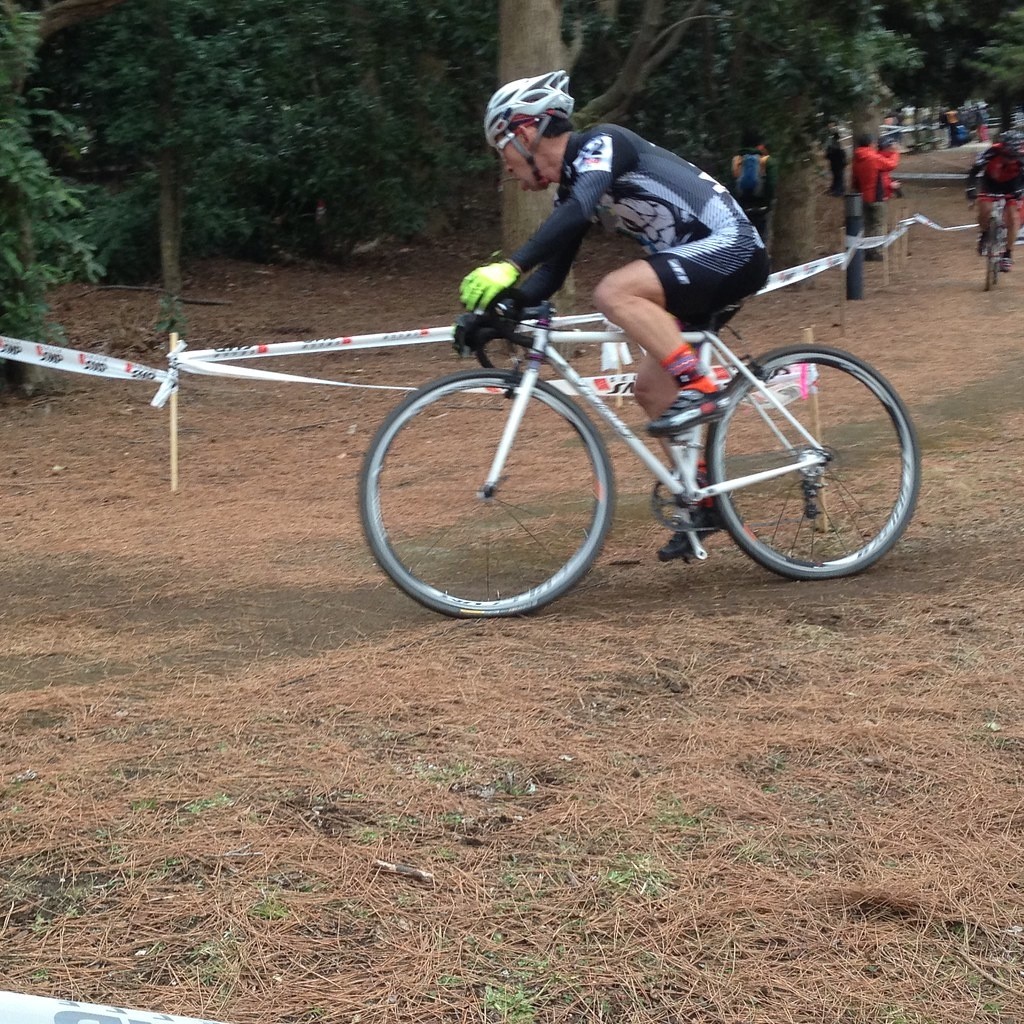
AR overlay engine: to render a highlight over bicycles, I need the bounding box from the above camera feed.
[355,252,922,617]
[968,191,1011,291]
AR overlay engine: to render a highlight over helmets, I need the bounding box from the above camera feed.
[483,69,576,147]
[1009,112,1024,128]
[878,136,899,149]
[1000,130,1024,153]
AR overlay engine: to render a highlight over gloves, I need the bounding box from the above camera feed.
[965,188,978,200]
[459,257,523,317]
[453,305,513,352]
[1014,189,1024,199]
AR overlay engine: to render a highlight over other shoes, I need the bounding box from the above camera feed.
[864,253,884,263]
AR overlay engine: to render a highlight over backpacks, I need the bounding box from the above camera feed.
[735,153,766,198]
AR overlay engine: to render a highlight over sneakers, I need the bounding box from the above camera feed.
[1000,253,1013,272]
[978,239,992,255]
[645,375,730,436]
[659,497,726,563]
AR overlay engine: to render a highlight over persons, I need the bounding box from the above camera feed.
[851,135,899,261]
[964,129,1024,270]
[827,133,847,197]
[940,102,989,144]
[732,144,777,243]
[878,135,902,234]
[452,70,771,561]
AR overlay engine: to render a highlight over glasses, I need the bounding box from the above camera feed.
[494,119,540,163]
